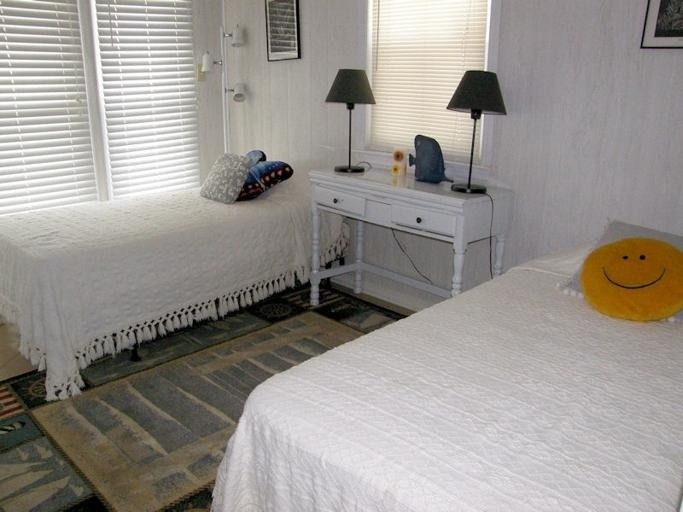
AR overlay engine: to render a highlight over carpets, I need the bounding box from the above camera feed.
[0,285,409,511]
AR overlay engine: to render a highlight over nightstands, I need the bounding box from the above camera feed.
[305,167,516,307]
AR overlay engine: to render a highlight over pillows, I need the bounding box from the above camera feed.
[561,215,682,325]
[199,149,294,204]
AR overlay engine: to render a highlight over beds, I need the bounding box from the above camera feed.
[220,231,683,512]
[0,172,347,401]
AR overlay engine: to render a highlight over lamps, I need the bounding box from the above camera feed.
[198,21,249,102]
[447,69,507,195]
[324,66,377,174]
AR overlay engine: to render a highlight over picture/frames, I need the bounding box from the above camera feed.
[639,0,683,48]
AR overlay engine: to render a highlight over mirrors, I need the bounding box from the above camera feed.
[264,0,301,61]
